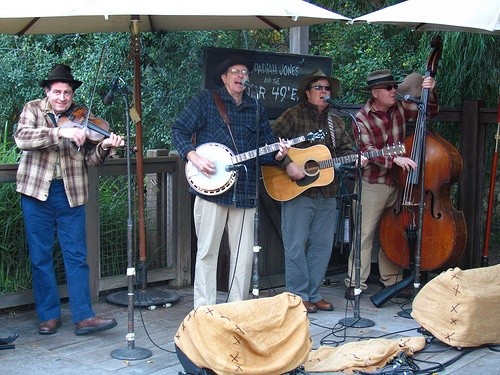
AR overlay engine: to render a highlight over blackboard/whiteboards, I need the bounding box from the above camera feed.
[200,47,332,120]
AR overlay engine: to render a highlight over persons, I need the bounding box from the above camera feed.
[171,56,290,310]
[270,68,368,312]
[344,68,439,300]
[14,64,125,334]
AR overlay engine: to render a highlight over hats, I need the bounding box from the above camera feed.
[296,68,339,102]
[40,64,83,91]
[213,52,253,87]
[309,85,330,91]
[363,69,402,90]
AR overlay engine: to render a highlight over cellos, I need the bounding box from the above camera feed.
[378,35,468,273]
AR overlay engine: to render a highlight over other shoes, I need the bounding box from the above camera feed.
[76,316,117,335]
[395,288,413,297]
[345,286,354,300]
[40,319,61,334]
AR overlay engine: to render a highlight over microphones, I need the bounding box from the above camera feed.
[404,95,424,104]
[241,79,260,94]
[224,164,245,172]
[324,95,341,110]
[104,74,119,105]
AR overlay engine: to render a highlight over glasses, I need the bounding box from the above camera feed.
[375,84,399,90]
[227,68,248,76]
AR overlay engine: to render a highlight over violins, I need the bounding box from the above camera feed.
[57,106,141,154]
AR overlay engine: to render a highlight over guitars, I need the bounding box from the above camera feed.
[183,128,328,194]
[258,140,409,203]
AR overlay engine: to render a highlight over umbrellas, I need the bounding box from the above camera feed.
[347,0,500,267]
[0,0,353,260]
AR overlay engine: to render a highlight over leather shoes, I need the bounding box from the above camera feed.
[315,299,334,311]
[303,301,317,312]
